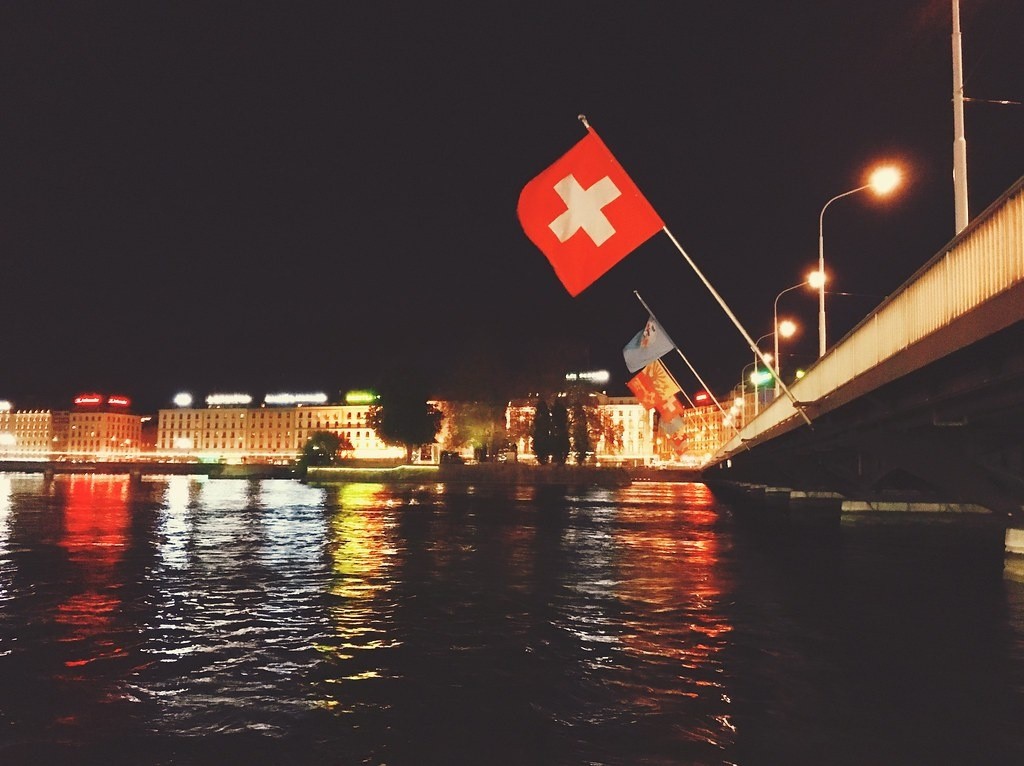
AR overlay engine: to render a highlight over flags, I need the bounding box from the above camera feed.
[626,361,681,410]
[653,396,689,457]
[622,313,677,374]
[515,123,666,298]
